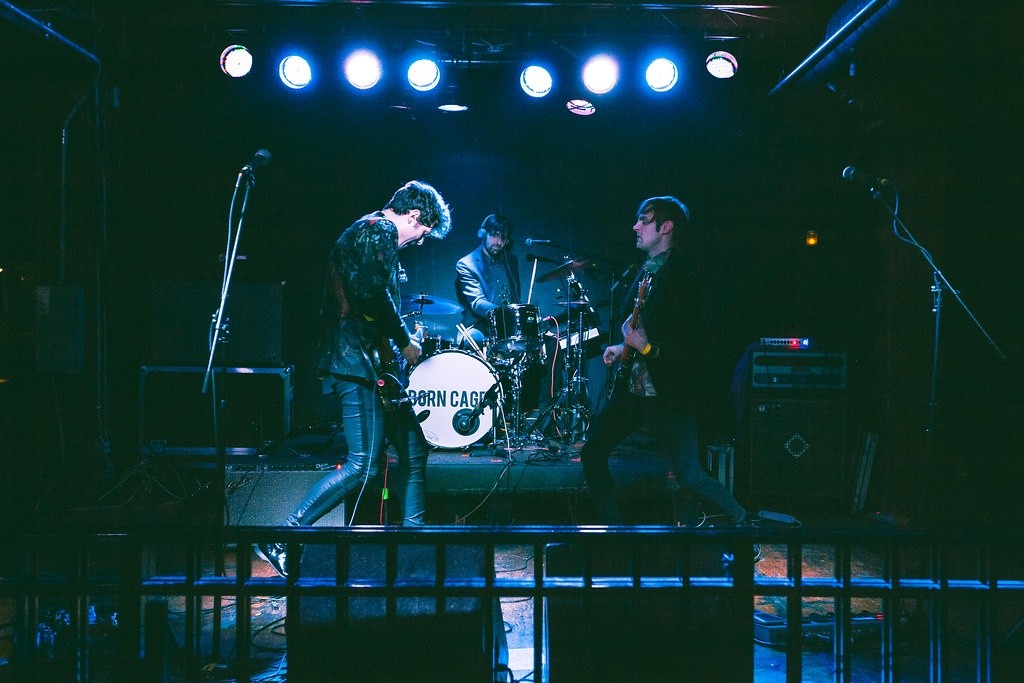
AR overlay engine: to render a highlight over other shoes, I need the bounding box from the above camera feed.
[737,513,762,562]
[255,541,287,579]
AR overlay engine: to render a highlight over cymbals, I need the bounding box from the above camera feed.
[534,252,596,285]
[552,299,592,307]
[401,292,465,317]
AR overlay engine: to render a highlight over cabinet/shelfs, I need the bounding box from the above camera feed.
[139,359,296,455]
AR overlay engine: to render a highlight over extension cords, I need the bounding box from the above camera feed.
[758,510,795,523]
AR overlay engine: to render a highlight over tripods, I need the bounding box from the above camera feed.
[469,244,605,458]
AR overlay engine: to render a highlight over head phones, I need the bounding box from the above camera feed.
[476,214,511,246]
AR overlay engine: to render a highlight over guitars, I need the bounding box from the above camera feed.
[373,318,429,415]
[605,268,657,404]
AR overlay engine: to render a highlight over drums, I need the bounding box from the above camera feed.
[487,302,540,342]
[405,347,504,451]
[406,334,454,377]
[488,338,541,374]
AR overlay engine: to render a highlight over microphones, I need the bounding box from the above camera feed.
[842,165,891,187]
[526,238,554,245]
[238,148,271,178]
[458,415,471,431]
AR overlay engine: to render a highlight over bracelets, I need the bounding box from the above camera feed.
[401,341,412,351]
[642,343,651,355]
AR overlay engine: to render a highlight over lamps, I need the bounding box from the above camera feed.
[579,42,621,93]
[636,48,679,94]
[278,49,319,90]
[565,78,600,116]
[437,73,474,111]
[389,79,417,113]
[340,42,384,91]
[701,35,744,79]
[218,41,263,81]
[405,52,442,93]
[518,62,558,103]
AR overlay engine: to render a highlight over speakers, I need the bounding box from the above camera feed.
[224,464,353,527]
[746,395,850,513]
[545,543,754,683]
[285,544,508,682]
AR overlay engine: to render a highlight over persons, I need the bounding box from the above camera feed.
[254,180,451,579]
[455,214,564,410]
[582,195,761,562]
